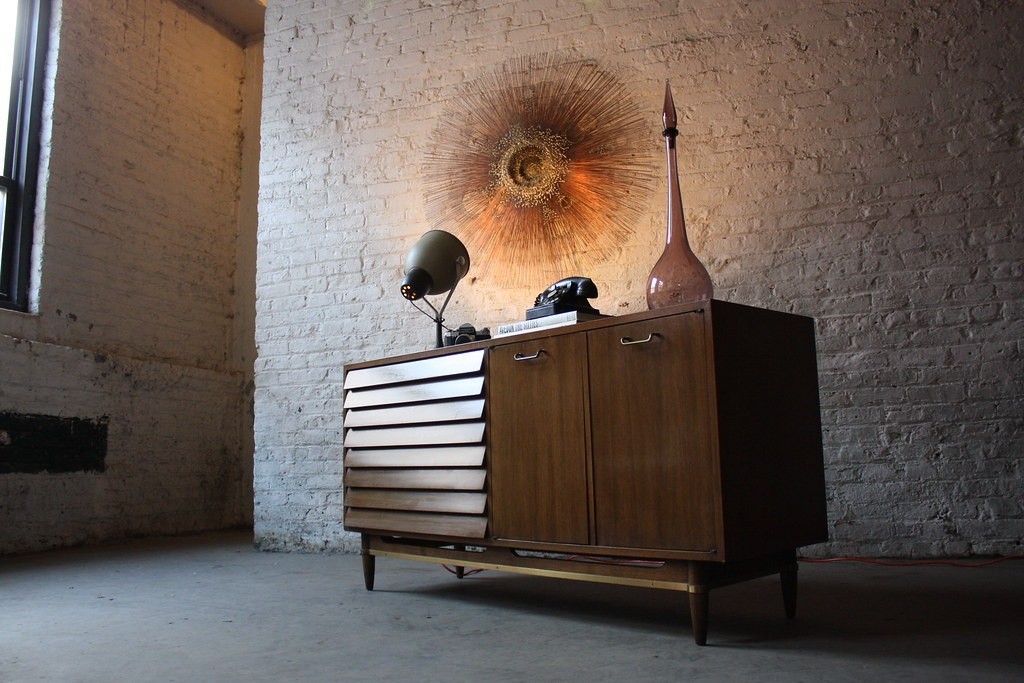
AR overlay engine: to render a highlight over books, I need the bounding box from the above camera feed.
[493,311,612,336]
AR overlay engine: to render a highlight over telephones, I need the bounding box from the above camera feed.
[525,277,600,321]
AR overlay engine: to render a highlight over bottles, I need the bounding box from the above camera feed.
[646,78,714,310]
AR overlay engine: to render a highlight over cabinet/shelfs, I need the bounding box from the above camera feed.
[341,299,827,647]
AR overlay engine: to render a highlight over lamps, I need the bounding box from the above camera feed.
[400,230,470,347]
